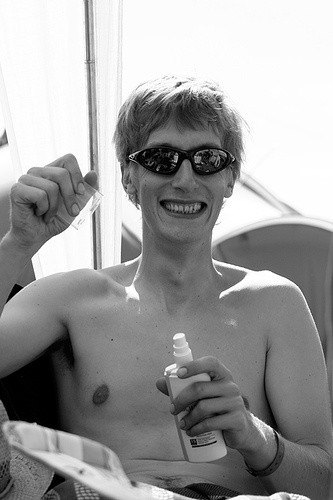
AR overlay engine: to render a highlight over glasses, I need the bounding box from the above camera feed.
[126,145,236,176]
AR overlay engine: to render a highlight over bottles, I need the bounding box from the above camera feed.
[164,333,228,466]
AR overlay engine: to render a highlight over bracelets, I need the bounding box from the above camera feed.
[244,429,285,476]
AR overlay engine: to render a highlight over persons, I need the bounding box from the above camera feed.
[0,75,333,500]
